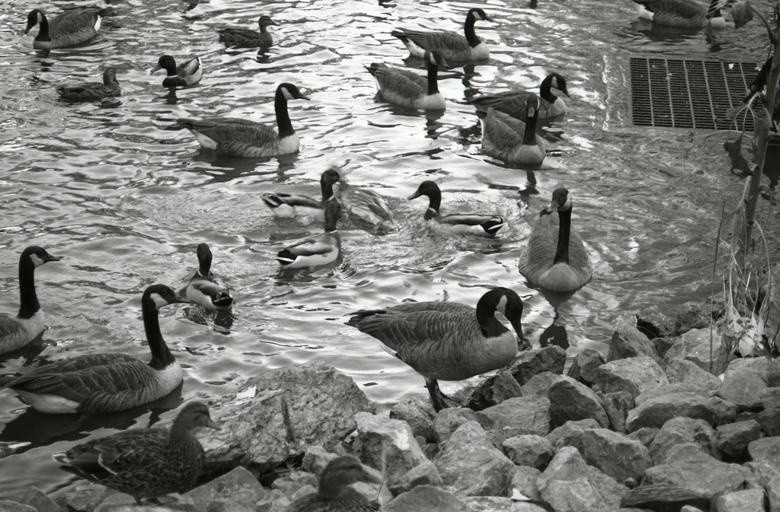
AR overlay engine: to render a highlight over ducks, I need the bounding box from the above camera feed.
[2,5,596,511]
[633,1,737,30]
